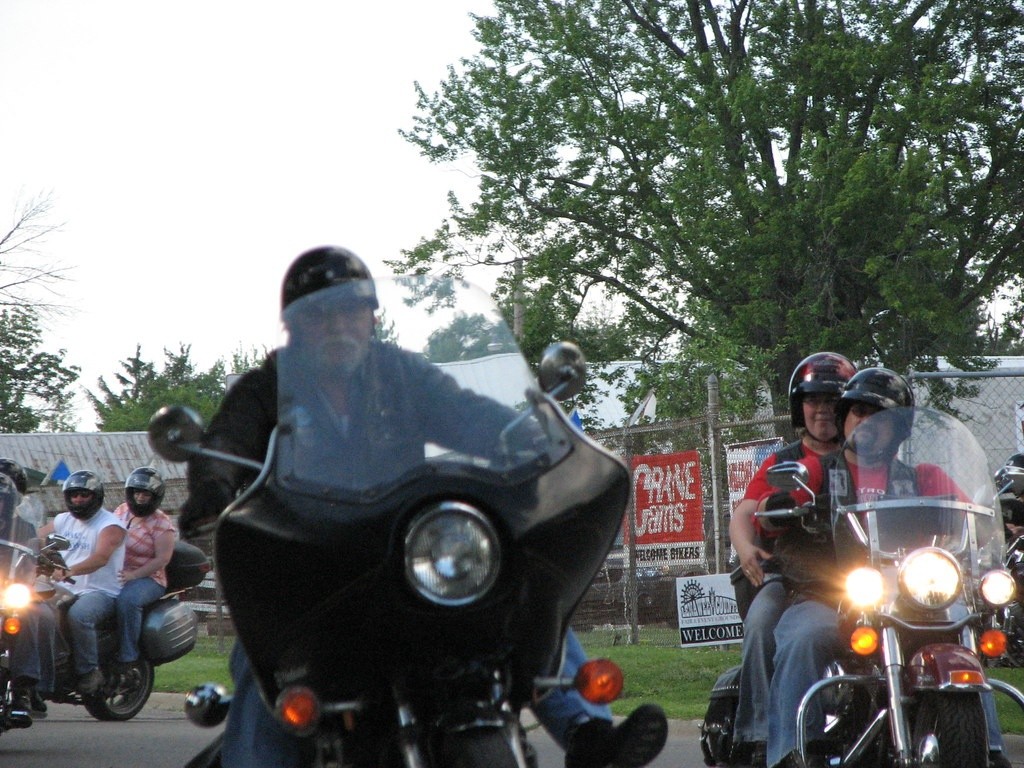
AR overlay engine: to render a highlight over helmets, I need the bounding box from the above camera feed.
[282,246,379,319]
[848,405,891,420]
[0,472,19,498]
[124,466,165,517]
[841,366,916,435]
[788,352,856,428]
[1,458,28,494]
[61,470,103,520]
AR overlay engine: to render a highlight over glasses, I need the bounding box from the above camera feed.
[68,490,92,498]
[801,396,838,406]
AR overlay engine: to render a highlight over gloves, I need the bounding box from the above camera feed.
[919,495,963,535]
[527,431,567,464]
[178,479,236,530]
[757,490,798,533]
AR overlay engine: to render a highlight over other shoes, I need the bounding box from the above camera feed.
[9,687,33,714]
[750,741,767,768]
[30,687,48,712]
[779,749,805,768]
[74,668,106,695]
[108,652,143,675]
[989,750,1012,768]
[565,703,668,768]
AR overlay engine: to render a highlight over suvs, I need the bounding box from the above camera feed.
[567,501,735,628]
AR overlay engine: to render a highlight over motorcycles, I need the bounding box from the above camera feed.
[0,485,212,733]
[696,406,1023,768]
[149,275,622,768]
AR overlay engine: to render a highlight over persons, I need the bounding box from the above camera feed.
[729,351,1024,767]
[35,470,127,693]
[0,459,67,713]
[113,467,174,669]
[178,247,669,768]
